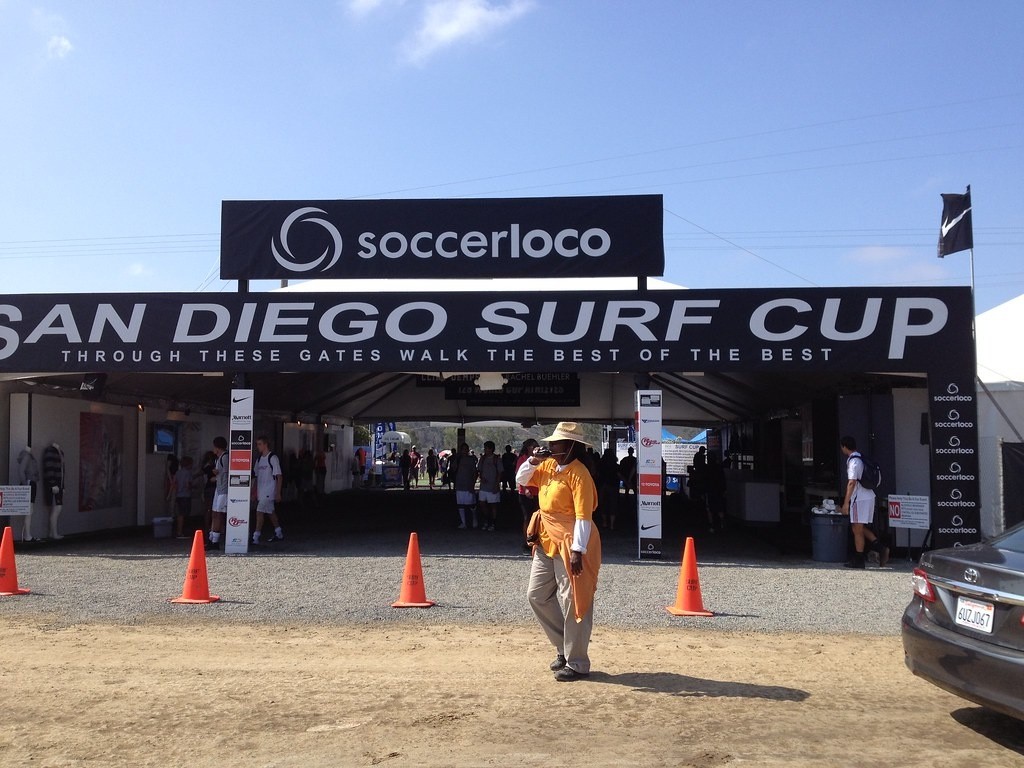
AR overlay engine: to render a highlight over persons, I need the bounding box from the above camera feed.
[694,446,724,534]
[446,443,479,529]
[353,448,366,488]
[516,438,540,556]
[390,445,456,492]
[478,441,504,531]
[515,421,601,682]
[586,447,617,530]
[621,447,636,495]
[289,448,327,496]
[165,436,284,551]
[299,451,319,511]
[19,443,64,542]
[502,445,517,491]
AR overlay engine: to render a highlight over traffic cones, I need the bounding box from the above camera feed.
[171,529,220,604]
[665,537,713,617]
[0,527,30,595]
[391,532,436,608]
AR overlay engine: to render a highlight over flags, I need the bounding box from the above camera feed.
[839,436,890,568]
[937,191,973,259]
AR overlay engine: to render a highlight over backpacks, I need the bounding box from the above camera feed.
[847,451,887,489]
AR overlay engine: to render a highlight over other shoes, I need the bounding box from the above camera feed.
[458,524,465,528]
[721,523,726,529]
[710,528,717,534]
[203,540,221,551]
[173,534,192,540]
[881,548,890,566]
[267,535,285,542]
[844,561,866,567]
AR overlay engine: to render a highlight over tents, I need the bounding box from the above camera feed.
[662,427,707,492]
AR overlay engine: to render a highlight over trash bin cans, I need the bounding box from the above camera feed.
[810,512,849,563]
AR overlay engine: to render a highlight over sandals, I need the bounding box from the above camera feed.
[480,524,496,532]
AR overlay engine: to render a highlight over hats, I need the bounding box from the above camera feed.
[412,445,417,449]
[539,422,592,448]
[700,447,706,450]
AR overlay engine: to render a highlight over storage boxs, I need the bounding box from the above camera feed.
[153,515,175,538]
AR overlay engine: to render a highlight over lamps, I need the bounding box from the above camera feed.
[138,404,145,412]
[341,424,345,428]
[324,421,329,428]
[297,418,302,427]
[183,409,191,416]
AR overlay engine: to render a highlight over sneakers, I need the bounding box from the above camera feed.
[550,655,566,671]
[522,547,532,556]
[555,666,590,681]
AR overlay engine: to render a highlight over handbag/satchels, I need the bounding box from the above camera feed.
[439,458,444,465]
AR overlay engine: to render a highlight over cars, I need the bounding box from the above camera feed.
[900,520,1024,722]
